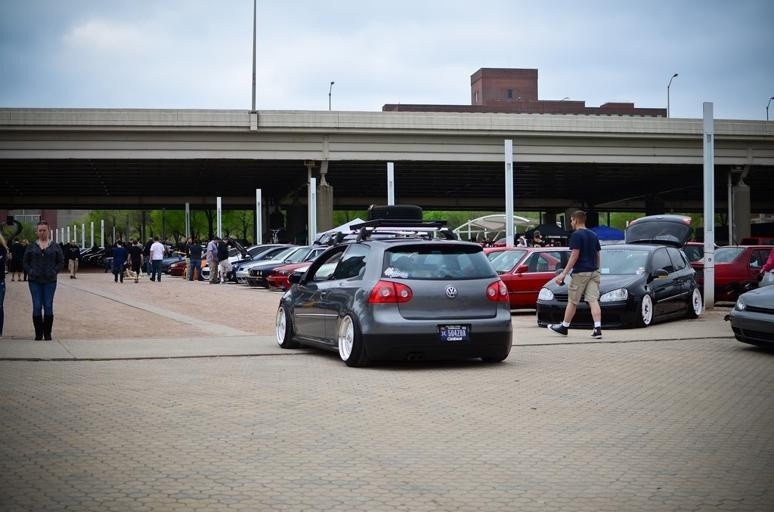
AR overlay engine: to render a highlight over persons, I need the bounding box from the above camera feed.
[547,211,602,339]
[750,252,758,267]
[0,235,9,336]
[22,221,65,341]
[758,247,774,274]
[470,237,495,248]
[92,233,235,283]
[514,227,557,248]
[8,238,80,281]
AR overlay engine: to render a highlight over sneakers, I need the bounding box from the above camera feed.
[591,327,602,338]
[548,324,568,336]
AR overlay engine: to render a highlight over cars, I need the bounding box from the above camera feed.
[536,214,702,328]
[680,241,774,348]
[276,204,513,367]
[77,247,150,272]
[481,242,573,310]
[158,235,332,292]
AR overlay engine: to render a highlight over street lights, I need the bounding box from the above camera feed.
[666,73,678,117]
[328,81,334,112]
[766,96,774,121]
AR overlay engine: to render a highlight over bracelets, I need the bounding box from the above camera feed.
[596,268,599,270]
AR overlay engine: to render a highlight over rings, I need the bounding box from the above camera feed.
[560,281,562,283]
